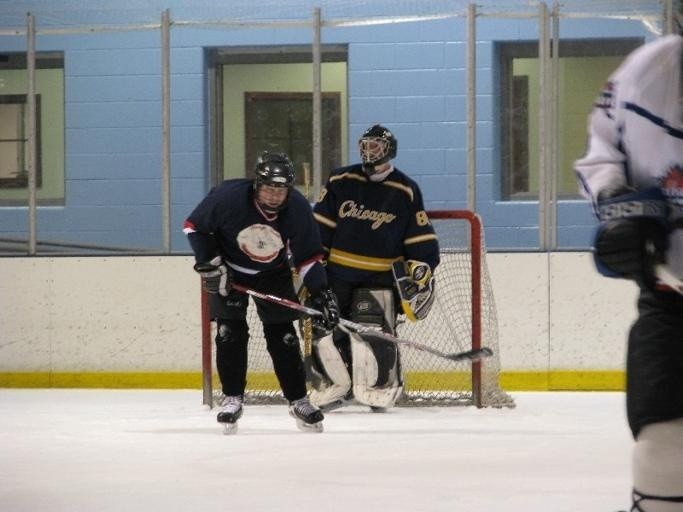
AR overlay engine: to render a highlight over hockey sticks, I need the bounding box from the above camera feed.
[230,285,492,361]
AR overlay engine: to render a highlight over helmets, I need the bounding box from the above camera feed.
[359,124,398,166]
[251,150,295,192]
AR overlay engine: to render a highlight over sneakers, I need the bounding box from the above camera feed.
[214,395,244,422]
[287,398,324,423]
[318,389,355,408]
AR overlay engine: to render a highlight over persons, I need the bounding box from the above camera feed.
[570,5,681,512]
[293,122,441,413]
[181,150,342,424]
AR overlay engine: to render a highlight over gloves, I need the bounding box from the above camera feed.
[193,263,231,297]
[307,289,339,331]
[593,185,673,279]
[403,262,434,320]
